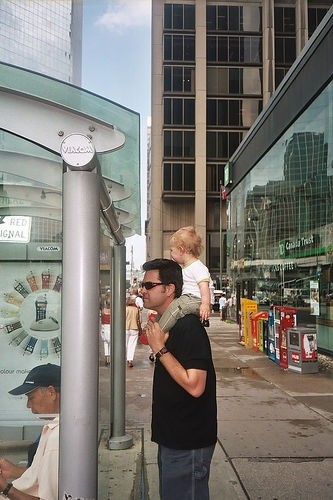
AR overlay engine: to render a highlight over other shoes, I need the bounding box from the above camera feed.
[128,362,134,367]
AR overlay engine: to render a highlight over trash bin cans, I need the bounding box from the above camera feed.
[287,328,318,373]
[222,306,227,320]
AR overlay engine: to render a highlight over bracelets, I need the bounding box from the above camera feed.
[3,483,12,495]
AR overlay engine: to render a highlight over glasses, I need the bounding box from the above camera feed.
[141,281,168,290]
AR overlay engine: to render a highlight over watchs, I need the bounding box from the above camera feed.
[155,347,168,359]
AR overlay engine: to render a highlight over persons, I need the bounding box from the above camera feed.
[149,225,214,333]
[219,294,229,321]
[101,293,112,365]
[140,257,219,500]
[0,364,60,500]
[125,285,144,367]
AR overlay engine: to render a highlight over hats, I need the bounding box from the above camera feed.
[8,363,61,396]
